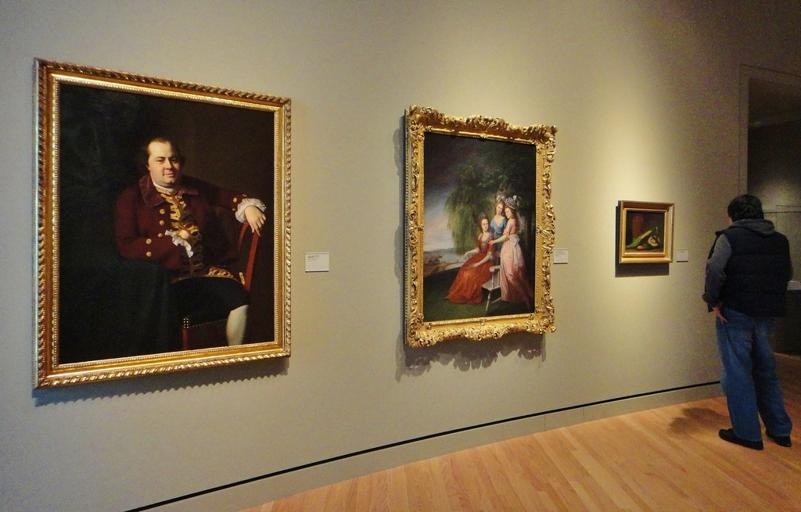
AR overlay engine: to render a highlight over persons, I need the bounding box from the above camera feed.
[109,134,269,347]
[487,203,534,313]
[702,193,795,449]
[486,198,507,266]
[446,213,497,306]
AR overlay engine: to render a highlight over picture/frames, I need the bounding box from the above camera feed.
[619,201,674,264]
[402,105,555,347]
[33,58,290,389]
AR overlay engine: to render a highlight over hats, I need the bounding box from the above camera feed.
[495,191,519,210]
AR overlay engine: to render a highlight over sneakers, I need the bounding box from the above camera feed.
[766,429,793,448]
[719,428,765,451]
